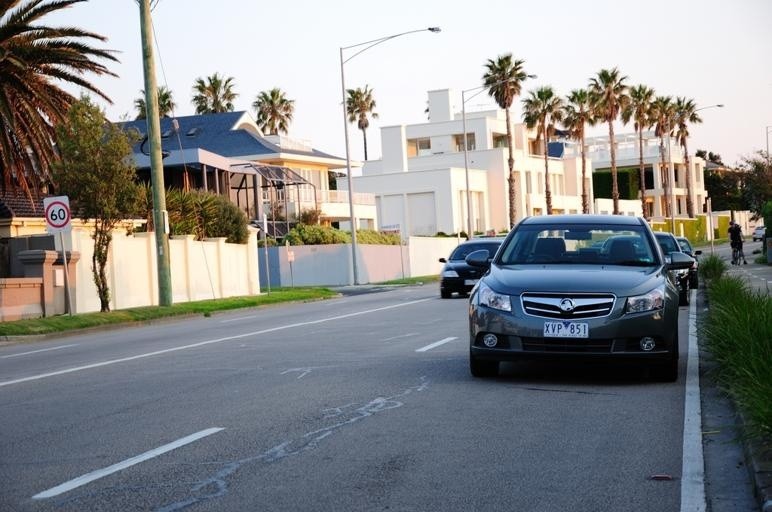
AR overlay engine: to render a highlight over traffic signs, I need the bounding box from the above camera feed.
[42,195,74,234]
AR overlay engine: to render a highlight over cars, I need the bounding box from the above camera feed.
[752,225,765,242]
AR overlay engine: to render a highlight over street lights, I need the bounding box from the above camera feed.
[667,104,725,234]
[462,74,537,238]
[340,27,441,284]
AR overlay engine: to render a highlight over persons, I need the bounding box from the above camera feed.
[727,220,747,264]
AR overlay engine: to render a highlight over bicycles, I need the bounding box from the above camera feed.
[727,240,745,267]
